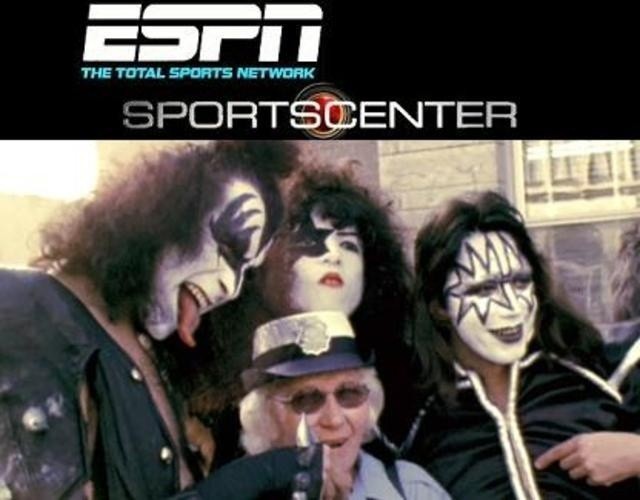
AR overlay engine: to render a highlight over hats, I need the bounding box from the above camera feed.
[241,307,380,387]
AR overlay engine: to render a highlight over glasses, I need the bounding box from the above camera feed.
[267,383,371,416]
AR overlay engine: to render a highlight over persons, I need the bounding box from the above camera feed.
[1,139,355,499]
[398,184,639,497]
[234,308,454,498]
[171,158,448,461]
